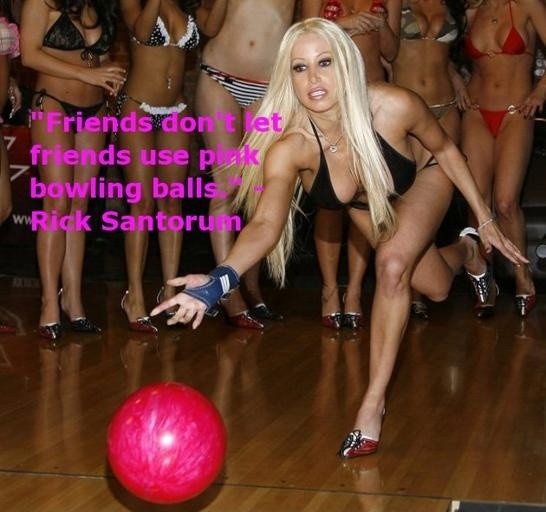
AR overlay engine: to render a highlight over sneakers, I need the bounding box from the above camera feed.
[0,316,17,337]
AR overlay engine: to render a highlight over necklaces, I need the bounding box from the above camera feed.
[492,19,498,24]
[310,115,343,153]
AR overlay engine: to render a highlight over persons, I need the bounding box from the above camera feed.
[300,0,402,330]
[19,0,128,341]
[0,0,23,334]
[30,323,532,505]
[150,17,530,459]
[193,1,296,331]
[391,0,462,319]
[447,0,546,320]
[116,0,202,334]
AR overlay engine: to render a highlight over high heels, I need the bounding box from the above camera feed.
[38,301,63,342]
[156,287,186,325]
[474,282,500,319]
[250,303,284,321]
[515,279,536,316]
[321,294,342,331]
[337,406,387,458]
[119,290,158,335]
[459,227,492,301]
[218,300,264,333]
[59,288,104,334]
[343,293,364,330]
[410,299,429,318]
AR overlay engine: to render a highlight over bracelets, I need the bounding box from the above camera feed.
[476,218,494,230]
